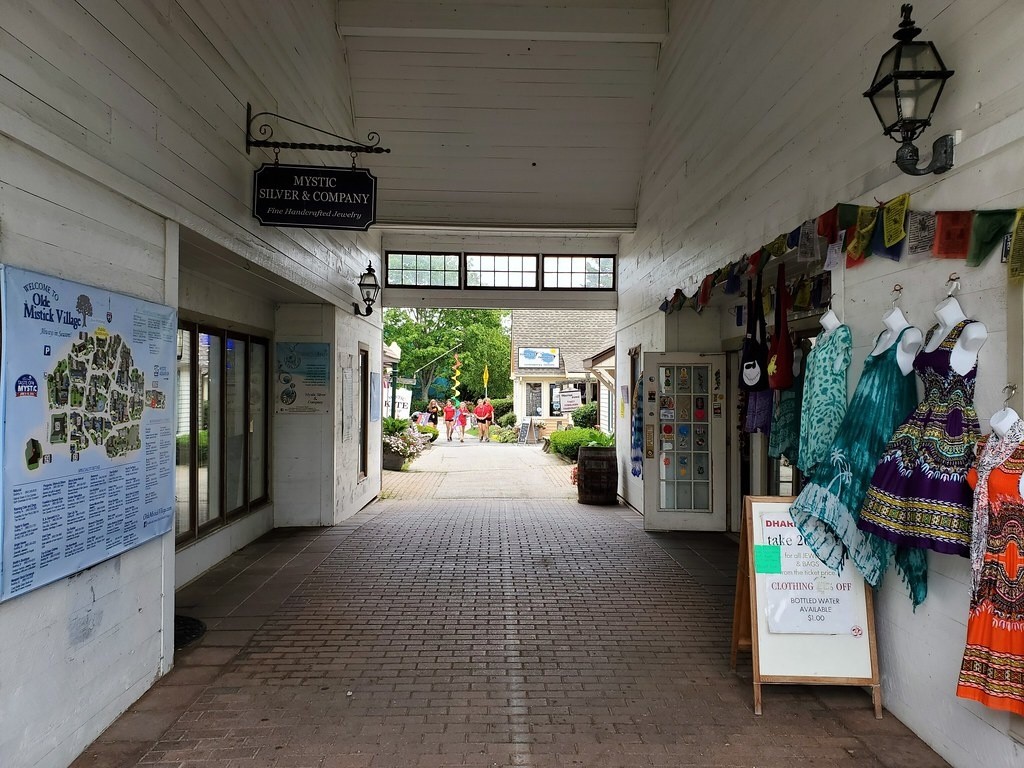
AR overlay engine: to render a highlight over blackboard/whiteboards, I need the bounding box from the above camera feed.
[734,495,879,687]
[518,418,537,443]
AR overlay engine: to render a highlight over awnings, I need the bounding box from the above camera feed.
[583,345,615,393]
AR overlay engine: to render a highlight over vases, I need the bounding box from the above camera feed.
[383,452,405,471]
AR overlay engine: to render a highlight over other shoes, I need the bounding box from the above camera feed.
[450,437,452,441]
[480,436,483,442]
[485,438,489,442]
[460,438,464,442]
[447,439,449,441]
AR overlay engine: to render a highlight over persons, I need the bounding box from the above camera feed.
[790,295,1024,717]
[426,399,441,429]
[443,399,456,442]
[457,398,494,443]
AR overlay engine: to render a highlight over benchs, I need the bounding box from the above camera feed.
[542,421,572,454]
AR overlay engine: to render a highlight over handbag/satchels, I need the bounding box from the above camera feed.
[768,325,794,390]
[738,338,769,391]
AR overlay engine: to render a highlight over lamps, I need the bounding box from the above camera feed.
[353,259,381,318]
[860,5,954,177]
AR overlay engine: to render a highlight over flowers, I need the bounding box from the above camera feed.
[382,431,411,455]
